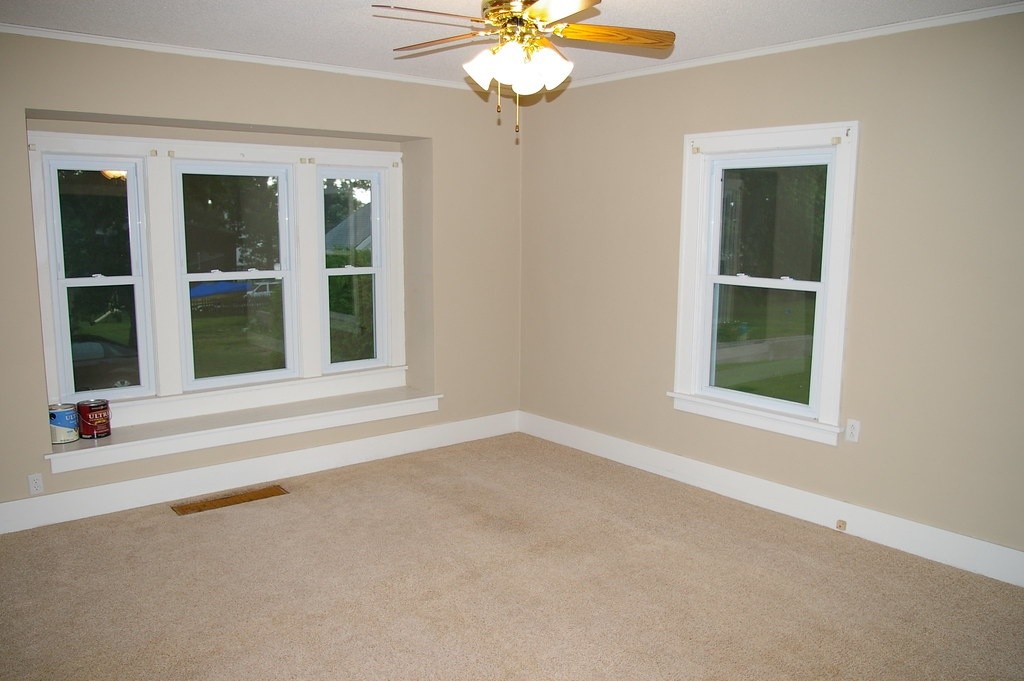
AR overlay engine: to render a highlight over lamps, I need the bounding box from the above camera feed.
[462,31,574,132]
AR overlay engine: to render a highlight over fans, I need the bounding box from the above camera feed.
[371,0,676,52]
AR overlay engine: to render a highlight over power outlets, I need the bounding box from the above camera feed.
[845,418,860,443]
[26,473,44,496]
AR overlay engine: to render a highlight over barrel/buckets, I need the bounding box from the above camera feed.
[77,399,112,439]
[48,403,78,445]
